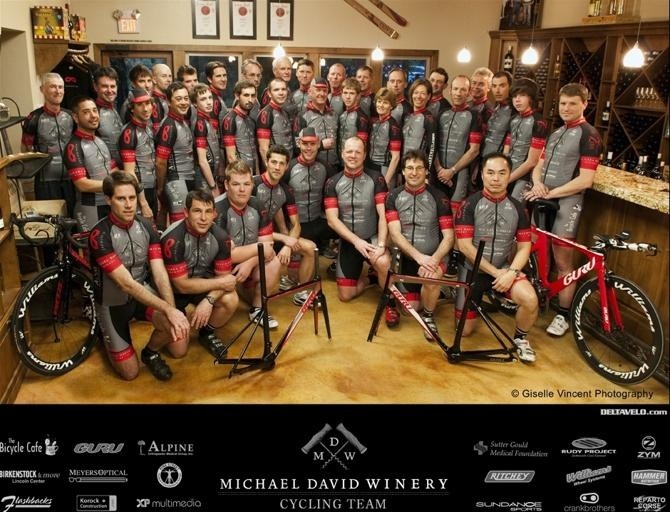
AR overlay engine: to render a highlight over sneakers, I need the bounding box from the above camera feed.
[322,246,338,259]
[385,299,400,328]
[197,333,227,359]
[327,260,337,275]
[141,349,172,381]
[546,314,569,337]
[422,316,438,339]
[437,286,457,299]
[248,304,279,329]
[512,338,537,362]
[279,274,321,310]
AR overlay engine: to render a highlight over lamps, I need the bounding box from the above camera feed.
[114,8,144,34]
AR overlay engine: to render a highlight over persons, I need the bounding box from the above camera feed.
[20,56,602,382]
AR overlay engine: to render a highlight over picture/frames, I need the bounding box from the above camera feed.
[229,0,257,40]
[191,0,220,39]
[267,0,293,40]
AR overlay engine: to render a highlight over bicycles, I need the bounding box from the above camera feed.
[13,211,174,381]
[516,189,665,388]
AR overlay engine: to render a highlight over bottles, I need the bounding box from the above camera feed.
[599,152,670,182]
[588,0,625,17]
[0,208,4,231]
[634,87,665,108]
[602,101,610,127]
[549,99,556,118]
[503,46,513,74]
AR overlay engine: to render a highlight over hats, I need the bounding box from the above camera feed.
[310,76,329,88]
[128,89,154,103]
[298,127,319,141]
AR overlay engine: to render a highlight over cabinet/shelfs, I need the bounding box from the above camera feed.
[488,21,670,181]
[0,97,73,343]
[0,152,49,404]
